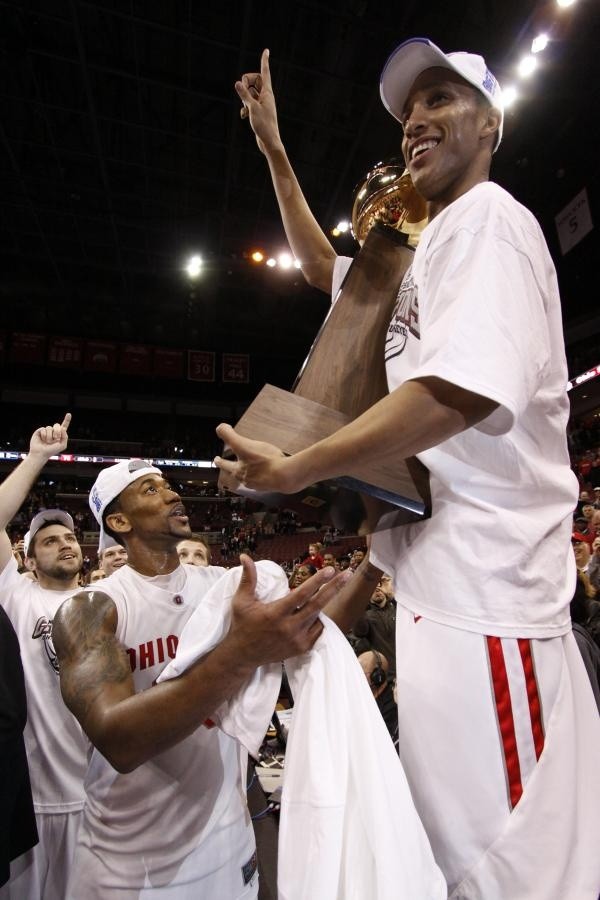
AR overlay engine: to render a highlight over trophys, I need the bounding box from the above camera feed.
[222,164,432,539]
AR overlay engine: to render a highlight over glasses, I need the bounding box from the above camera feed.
[572,541,581,546]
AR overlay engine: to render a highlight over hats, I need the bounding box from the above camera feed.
[88,460,163,555]
[594,487,600,490]
[379,38,505,154]
[24,509,74,558]
[571,531,592,543]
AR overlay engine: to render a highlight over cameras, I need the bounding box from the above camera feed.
[371,669,385,686]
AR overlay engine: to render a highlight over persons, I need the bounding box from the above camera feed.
[0,413,600,900]
[234,38,581,895]
